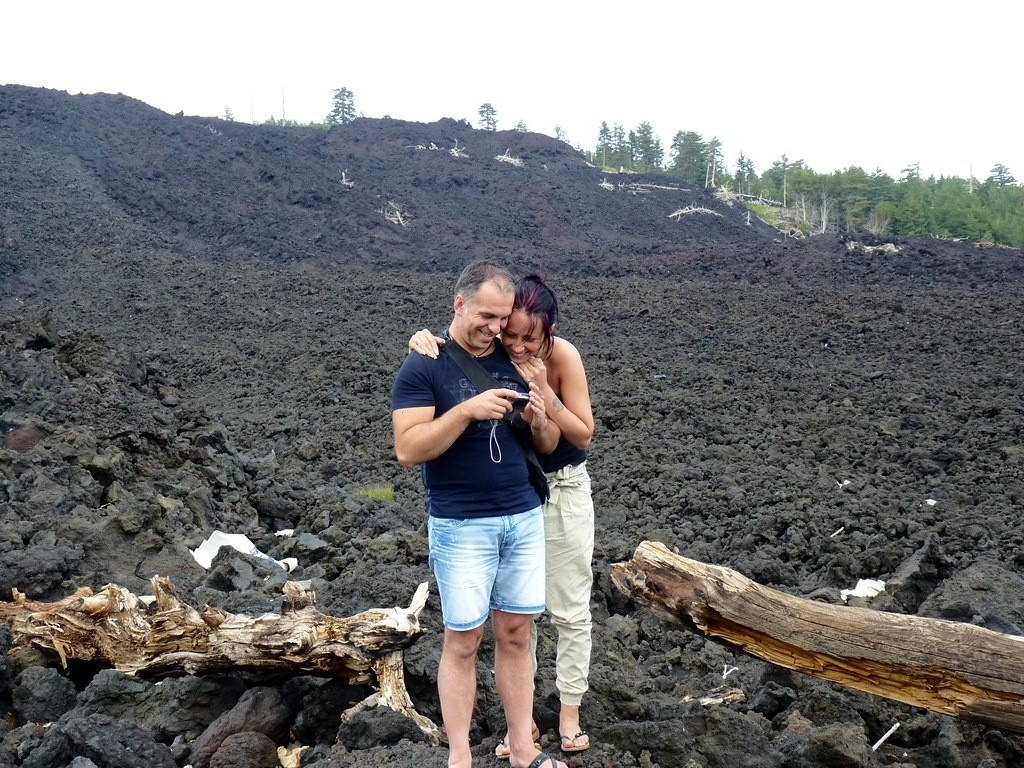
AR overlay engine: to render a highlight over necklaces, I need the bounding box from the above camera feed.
[448,329,494,358]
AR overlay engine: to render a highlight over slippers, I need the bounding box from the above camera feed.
[496,728,540,759]
[561,731,589,751]
[510,753,570,768]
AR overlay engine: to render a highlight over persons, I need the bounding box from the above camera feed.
[409,274,597,761]
[392,259,570,768]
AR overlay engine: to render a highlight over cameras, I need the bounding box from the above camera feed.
[503,393,530,411]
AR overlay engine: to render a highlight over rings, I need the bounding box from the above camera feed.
[416,339,422,346]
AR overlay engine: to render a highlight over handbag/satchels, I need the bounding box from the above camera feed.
[507,412,550,500]
